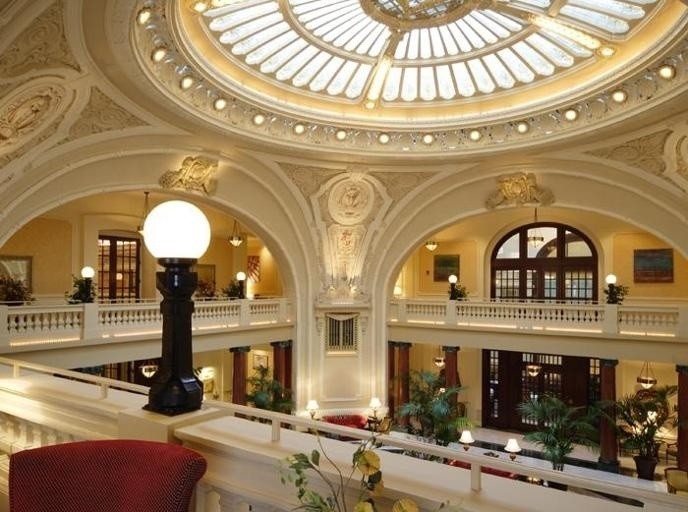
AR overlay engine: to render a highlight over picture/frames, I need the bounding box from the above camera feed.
[0,254,32,294]
[189,264,215,291]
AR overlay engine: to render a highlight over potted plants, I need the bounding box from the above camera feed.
[597,384,687,480]
[513,389,611,493]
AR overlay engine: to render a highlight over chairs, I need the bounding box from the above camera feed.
[6,438,208,512]
[665,441,677,466]
[615,419,635,457]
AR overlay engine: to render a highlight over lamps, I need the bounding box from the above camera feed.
[79,266,94,303]
[457,430,477,451]
[447,274,458,300]
[141,200,214,417]
[236,272,246,299]
[605,274,617,304]
[432,345,445,368]
[137,191,150,238]
[503,438,521,462]
[635,361,657,390]
[423,241,438,252]
[305,400,319,419]
[369,398,381,418]
[226,219,244,248]
[525,354,541,379]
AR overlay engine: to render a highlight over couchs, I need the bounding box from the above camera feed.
[321,415,367,442]
